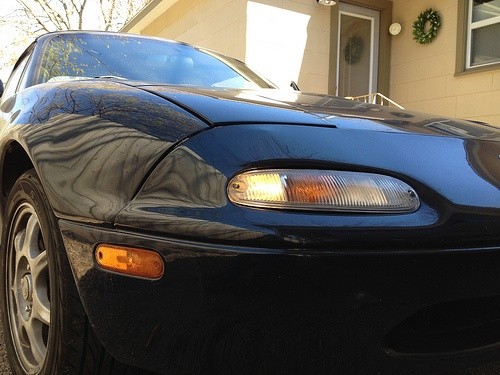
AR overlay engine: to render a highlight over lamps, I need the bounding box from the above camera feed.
[388,22,402,39]
[317,0,337,7]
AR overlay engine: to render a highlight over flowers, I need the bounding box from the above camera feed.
[344,35,365,65]
[412,8,441,44]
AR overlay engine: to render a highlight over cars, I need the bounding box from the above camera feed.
[0,28,500,375]
